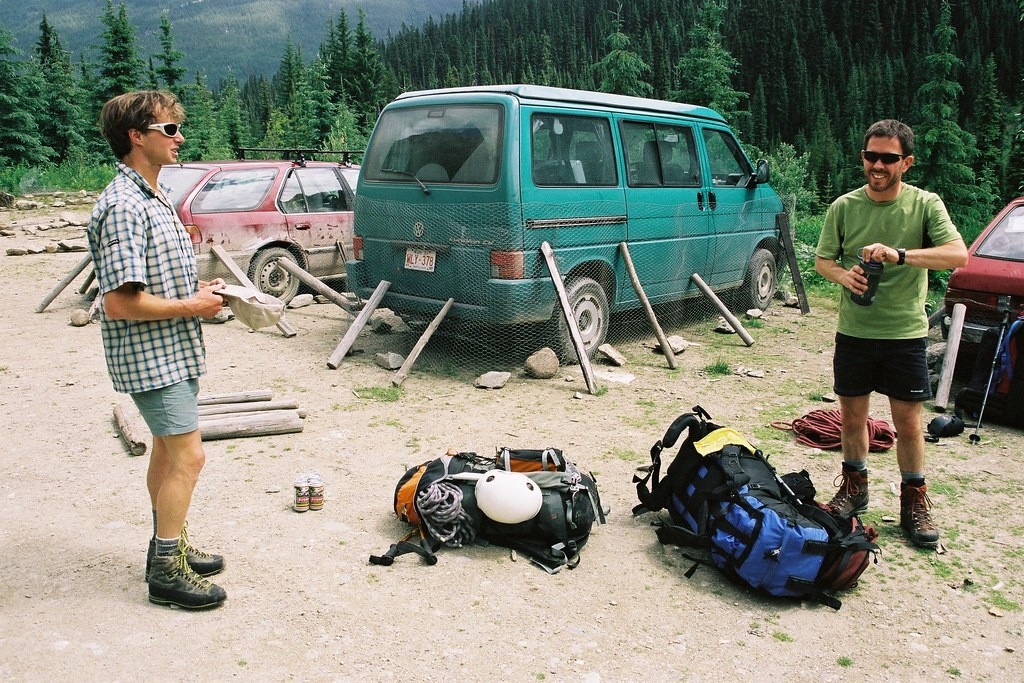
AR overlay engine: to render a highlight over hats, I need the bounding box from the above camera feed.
[213,284,286,330]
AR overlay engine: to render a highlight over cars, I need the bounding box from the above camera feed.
[939,197,1024,390]
[148,147,367,312]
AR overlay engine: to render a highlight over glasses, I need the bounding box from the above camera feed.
[863,151,905,164]
[147,123,181,137]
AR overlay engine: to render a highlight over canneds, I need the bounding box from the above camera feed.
[294,473,324,511]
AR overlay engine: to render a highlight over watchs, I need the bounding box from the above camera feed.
[896,248,906,265]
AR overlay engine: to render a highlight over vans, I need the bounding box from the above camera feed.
[344,82,791,365]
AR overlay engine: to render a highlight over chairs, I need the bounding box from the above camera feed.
[299,177,323,208]
[637,138,690,183]
[576,141,614,182]
[532,159,578,185]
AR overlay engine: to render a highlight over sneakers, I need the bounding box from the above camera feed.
[145,520,227,582]
[148,538,227,610]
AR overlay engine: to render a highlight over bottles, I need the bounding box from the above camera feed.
[309,476,323,510]
[294,479,309,511]
[850,246,884,307]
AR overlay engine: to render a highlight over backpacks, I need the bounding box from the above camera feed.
[631,405,882,611]
[990,316,1024,395]
[368,446,606,576]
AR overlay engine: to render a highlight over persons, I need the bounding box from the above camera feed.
[815,120,969,545]
[85,91,227,610]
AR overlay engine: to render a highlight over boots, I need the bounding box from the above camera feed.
[825,461,869,520]
[900,481,939,547]
